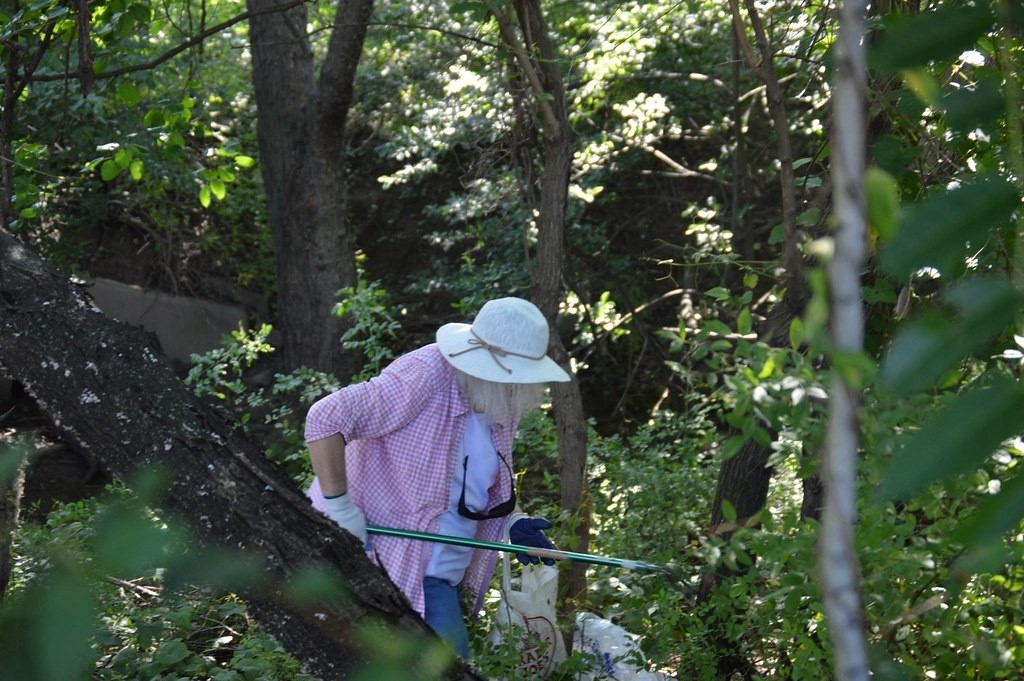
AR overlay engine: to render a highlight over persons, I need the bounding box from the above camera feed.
[303,296,572,673]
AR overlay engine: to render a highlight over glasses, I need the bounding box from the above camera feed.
[457,451,516,520]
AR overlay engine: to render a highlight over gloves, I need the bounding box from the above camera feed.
[509,517,561,565]
[323,491,368,548]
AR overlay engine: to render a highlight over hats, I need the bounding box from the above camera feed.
[436,296,571,383]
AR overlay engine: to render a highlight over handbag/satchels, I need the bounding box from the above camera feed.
[492,513,569,680]
